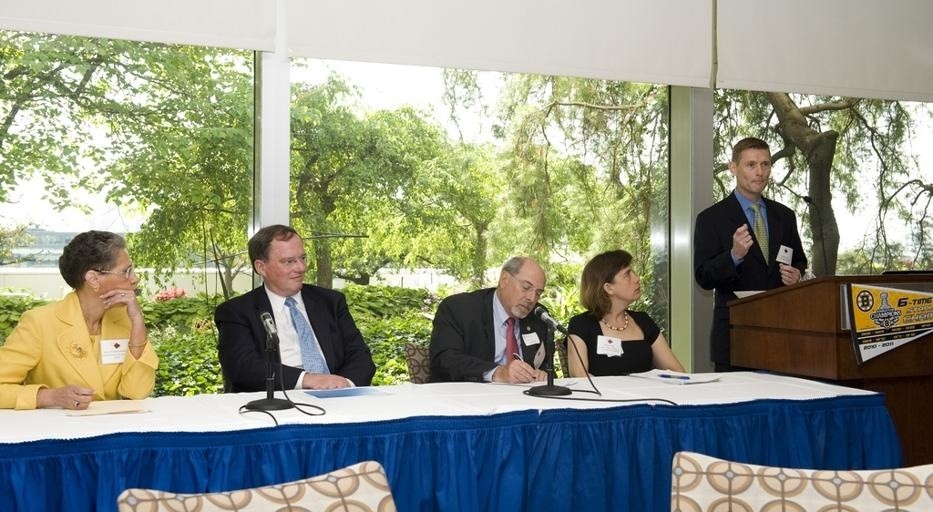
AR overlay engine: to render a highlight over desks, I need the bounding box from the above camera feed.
[0,370,884,512]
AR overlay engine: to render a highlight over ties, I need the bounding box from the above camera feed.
[748,203,768,263]
[285,298,328,374]
[506,317,519,363]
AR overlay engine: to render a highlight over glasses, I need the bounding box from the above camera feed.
[508,270,542,296]
[96,267,133,280]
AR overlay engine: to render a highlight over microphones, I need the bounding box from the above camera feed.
[534,304,570,337]
[257,301,282,346]
[803,195,831,273]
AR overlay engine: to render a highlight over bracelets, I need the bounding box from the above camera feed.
[126,342,150,349]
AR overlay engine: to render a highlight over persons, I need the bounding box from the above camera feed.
[561,249,687,378]
[691,136,808,374]
[213,224,378,393]
[0,230,161,411]
[424,254,558,385]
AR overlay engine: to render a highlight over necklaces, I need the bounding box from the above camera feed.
[598,308,629,332]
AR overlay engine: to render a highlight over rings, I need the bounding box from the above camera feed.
[120,292,125,298]
[71,400,79,409]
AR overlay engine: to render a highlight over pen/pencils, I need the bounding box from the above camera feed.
[658,375,690,379]
[512,353,536,381]
